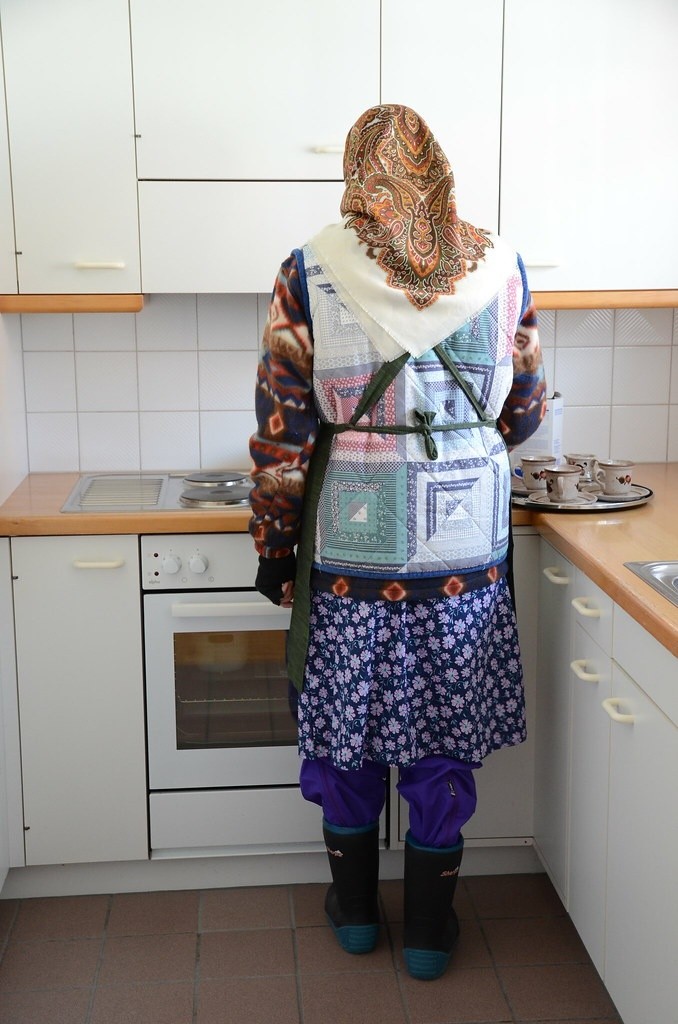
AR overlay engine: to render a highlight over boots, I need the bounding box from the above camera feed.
[402,828,464,980]
[322,816,380,954]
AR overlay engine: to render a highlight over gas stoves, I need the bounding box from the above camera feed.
[59,470,251,512]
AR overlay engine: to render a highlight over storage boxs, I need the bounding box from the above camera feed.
[508,387,562,477]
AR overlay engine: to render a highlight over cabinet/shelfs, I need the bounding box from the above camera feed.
[391,529,537,852]
[129,0,381,181]
[0,24,20,294]
[7,534,151,867]
[601,597,678,1023]
[499,0,677,292]
[1,0,143,294]
[562,564,616,992]
[532,535,572,925]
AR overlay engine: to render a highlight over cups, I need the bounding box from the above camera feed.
[563,453,601,481]
[542,464,582,502]
[511,455,556,490]
[595,459,635,495]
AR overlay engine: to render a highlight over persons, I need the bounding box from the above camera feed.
[250,105,547,980]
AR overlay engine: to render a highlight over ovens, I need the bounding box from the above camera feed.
[139,533,305,790]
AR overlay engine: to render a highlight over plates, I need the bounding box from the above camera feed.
[579,483,653,501]
[528,490,598,505]
[510,484,532,494]
